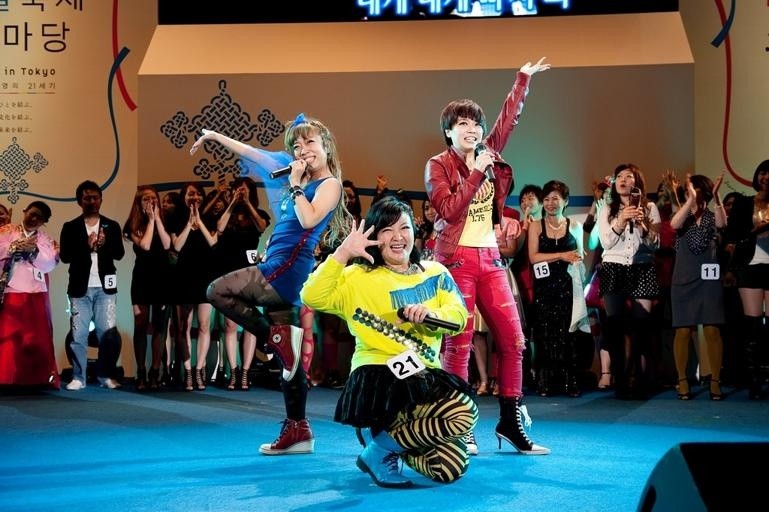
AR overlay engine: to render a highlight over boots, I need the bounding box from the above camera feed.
[741,312,769,400]
[356,426,374,448]
[464,431,478,455]
[495,397,551,455]
[356,430,412,488]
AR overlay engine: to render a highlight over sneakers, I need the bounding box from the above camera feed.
[97,376,122,389]
[539,386,547,397]
[477,383,490,395]
[66,379,86,390]
[320,372,345,389]
[570,392,579,397]
[264,324,304,382]
[492,384,500,396]
[259,417,314,455]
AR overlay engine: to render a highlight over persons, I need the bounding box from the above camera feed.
[1,201,64,393]
[1,202,13,224]
[123,175,258,395]
[58,179,125,391]
[297,196,480,488]
[187,111,356,459]
[422,57,554,457]
[470,161,769,402]
[299,181,436,390]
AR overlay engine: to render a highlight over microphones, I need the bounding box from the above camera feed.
[476,142,495,183]
[270,167,291,178]
[397,308,461,331]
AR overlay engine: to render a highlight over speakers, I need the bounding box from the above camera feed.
[637,442,768,512]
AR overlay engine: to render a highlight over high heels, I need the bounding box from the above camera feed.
[709,377,722,401]
[184,367,194,391]
[591,372,614,392]
[677,377,690,400]
[136,367,146,393]
[201,367,208,386]
[239,367,251,391]
[194,366,206,390]
[216,365,227,389]
[227,366,239,391]
[148,366,159,392]
